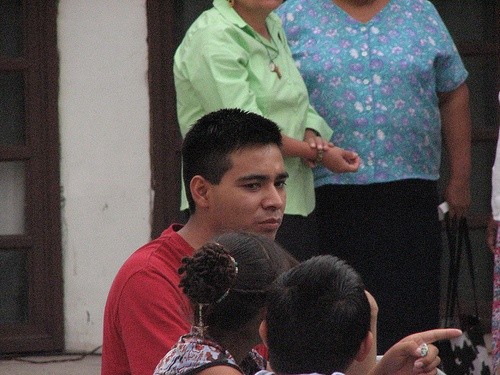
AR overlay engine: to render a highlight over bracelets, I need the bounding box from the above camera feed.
[316,149,326,164]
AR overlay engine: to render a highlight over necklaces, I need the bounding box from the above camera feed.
[254,25,281,79]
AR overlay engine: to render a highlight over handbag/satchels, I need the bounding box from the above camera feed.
[434,322,492,375]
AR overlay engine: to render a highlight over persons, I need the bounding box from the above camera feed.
[155,0,500,375]
[101,108,286,375]
